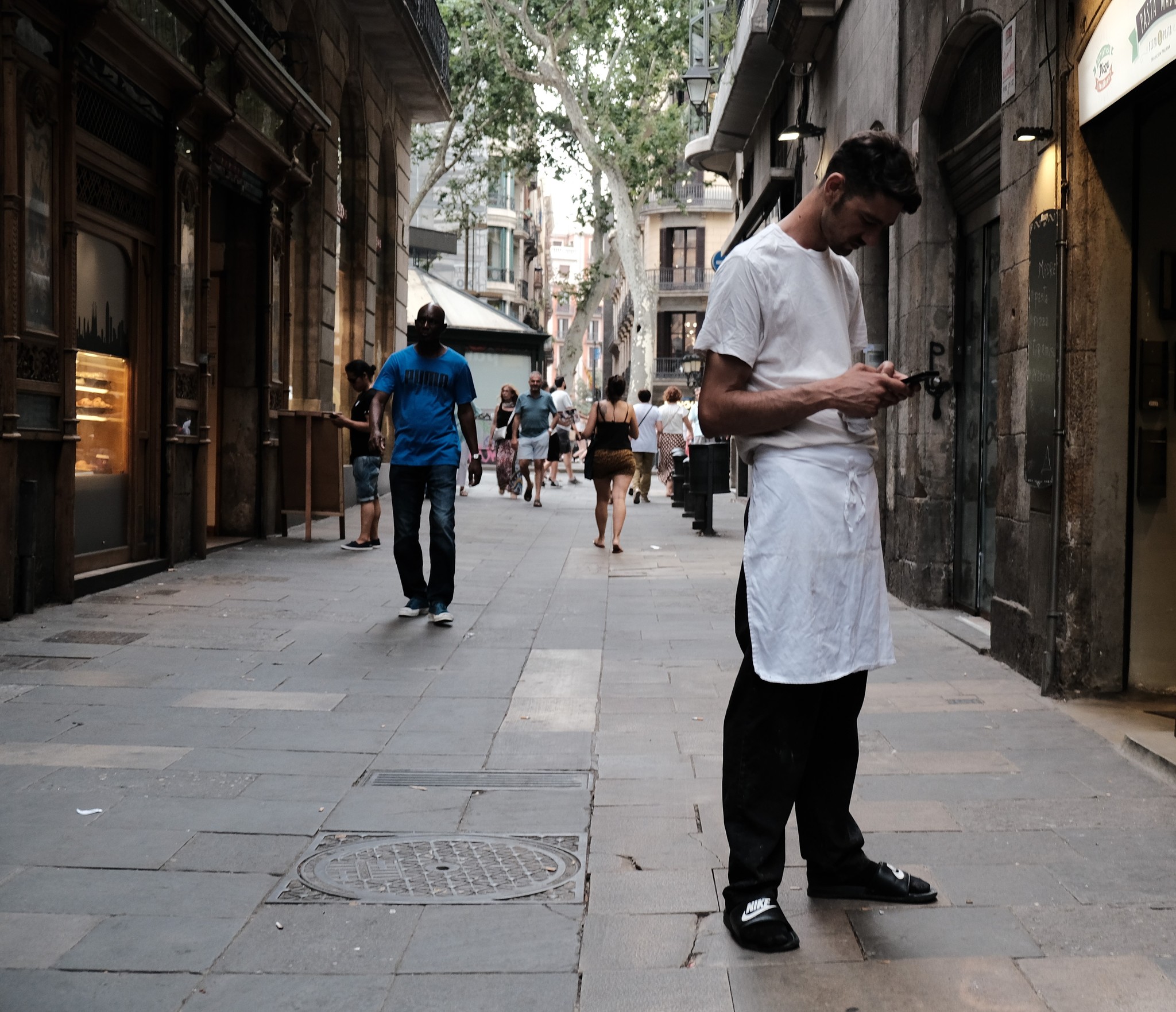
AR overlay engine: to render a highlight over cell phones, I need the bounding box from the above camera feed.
[901,369,940,384]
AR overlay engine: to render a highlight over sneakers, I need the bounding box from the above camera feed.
[399,589,430,616]
[371,539,381,548]
[341,540,373,550]
[427,601,453,622]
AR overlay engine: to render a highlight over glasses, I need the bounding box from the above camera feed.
[347,375,361,383]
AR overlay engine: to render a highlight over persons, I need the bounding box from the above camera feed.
[368,303,484,623]
[488,370,715,554]
[693,132,941,955]
[319,360,385,552]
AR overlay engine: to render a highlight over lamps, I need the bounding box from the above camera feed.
[1011,124,1055,145]
[680,349,704,388]
[777,120,829,144]
[675,54,716,124]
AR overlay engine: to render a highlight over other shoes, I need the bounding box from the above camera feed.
[542,481,545,487]
[550,482,557,486]
[569,479,581,485]
[633,489,641,504]
[628,487,634,496]
[499,489,505,494]
[460,489,468,496]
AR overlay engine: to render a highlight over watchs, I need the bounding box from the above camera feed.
[470,453,482,460]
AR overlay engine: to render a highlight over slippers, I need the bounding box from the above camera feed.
[723,884,800,953]
[524,483,534,501]
[594,539,605,548]
[808,858,936,903]
[533,500,542,507]
[612,548,623,554]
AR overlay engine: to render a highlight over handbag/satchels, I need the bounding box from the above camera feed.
[492,426,508,440]
